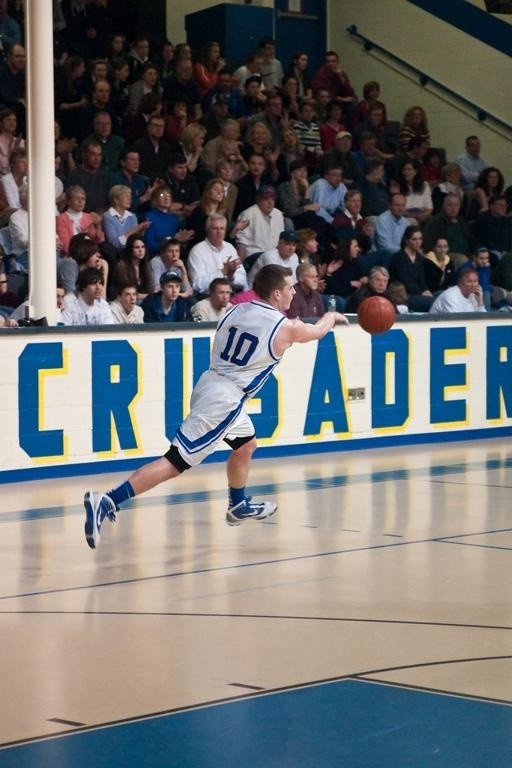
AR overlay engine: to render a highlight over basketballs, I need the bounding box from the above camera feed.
[359,296,394,332]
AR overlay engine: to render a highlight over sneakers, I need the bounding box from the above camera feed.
[225,495,279,528]
[82,490,120,550]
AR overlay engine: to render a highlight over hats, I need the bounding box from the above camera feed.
[256,184,277,198]
[244,76,262,89]
[280,230,300,243]
[159,270,183,288]
[336,130,353,140]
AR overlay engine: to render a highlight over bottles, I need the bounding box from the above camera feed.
[328,294,336,312]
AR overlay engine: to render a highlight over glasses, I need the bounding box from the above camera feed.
[158,192,172,198]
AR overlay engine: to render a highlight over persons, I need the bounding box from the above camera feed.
[84,264,350,549]
